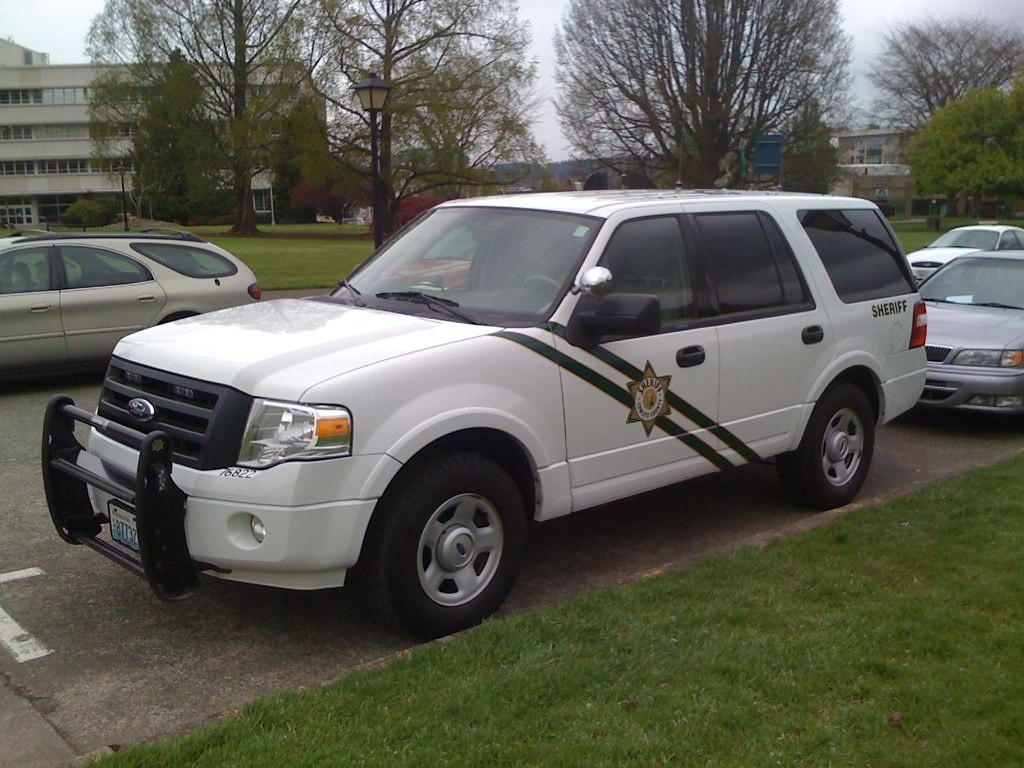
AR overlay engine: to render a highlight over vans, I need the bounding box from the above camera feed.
[0,228,260,380]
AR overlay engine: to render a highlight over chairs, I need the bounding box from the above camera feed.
[11,262,39,292]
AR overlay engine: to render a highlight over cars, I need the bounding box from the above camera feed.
[910,251,1023,417]
[907,222,1024,287]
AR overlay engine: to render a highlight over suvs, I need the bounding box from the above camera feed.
[40,184,931,636]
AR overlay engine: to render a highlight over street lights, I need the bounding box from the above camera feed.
[348,71,396,249]
